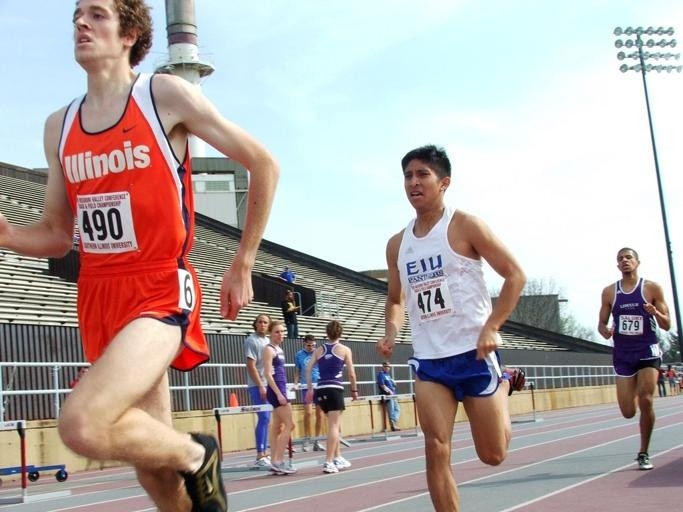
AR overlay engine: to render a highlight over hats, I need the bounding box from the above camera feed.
[383,361,392,367]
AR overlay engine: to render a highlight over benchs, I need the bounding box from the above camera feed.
[0,177,569,351]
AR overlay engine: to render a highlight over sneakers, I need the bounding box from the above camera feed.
[638,452,653,470]
[323,457,352,473]
[255,456,297,474]
[177,432,227,512]
[503,368,525,396]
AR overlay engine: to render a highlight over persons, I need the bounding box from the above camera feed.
[243,265,403,474]
[655,360,682,399]
[69,367,88,387]
[0,0,281,512]
[598,245,672,469]
[378,144,527,512]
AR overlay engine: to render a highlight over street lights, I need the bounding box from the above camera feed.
[612,25,683,369]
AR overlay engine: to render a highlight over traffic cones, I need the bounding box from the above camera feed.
[228,392,238,406]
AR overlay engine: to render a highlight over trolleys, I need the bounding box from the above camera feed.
[0,464,67,483]
